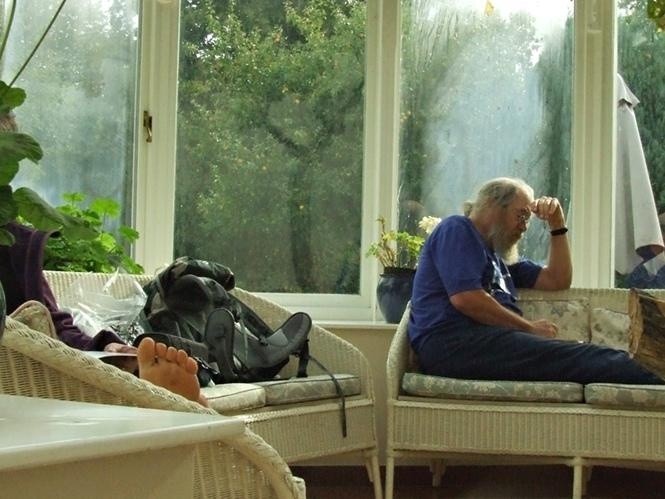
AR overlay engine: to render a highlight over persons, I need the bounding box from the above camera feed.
[0,109,213,410]
[406,178,664,383]
[621,212,665,287]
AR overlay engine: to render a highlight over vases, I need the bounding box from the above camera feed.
[377,267,416,324]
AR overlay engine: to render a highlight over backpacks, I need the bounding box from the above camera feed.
[134,257,313,398]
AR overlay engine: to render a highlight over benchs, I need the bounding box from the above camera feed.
[386,285,665,499]
[0,270,383,499]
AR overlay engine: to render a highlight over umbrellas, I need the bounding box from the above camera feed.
[565,73,664,277]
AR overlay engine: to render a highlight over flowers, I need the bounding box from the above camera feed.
[367,216,442,268]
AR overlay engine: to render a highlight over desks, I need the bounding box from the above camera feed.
[0,394,245,499]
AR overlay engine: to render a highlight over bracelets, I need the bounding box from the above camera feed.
[547,224,569,238]
